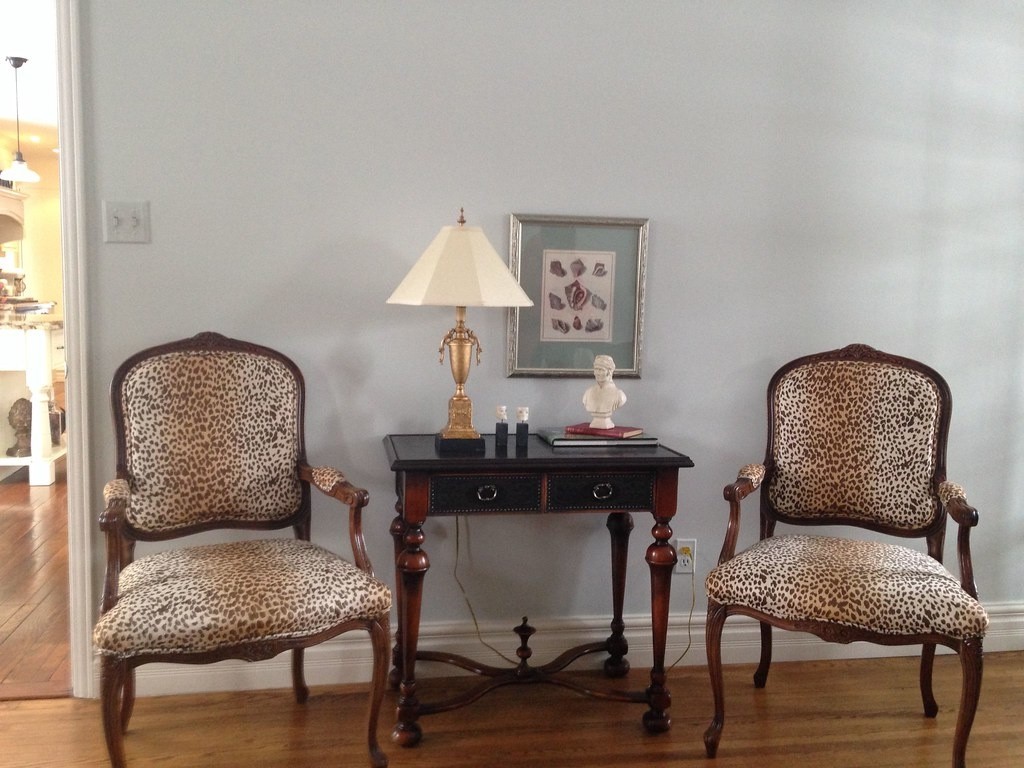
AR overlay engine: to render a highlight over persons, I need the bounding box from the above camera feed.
[581,355,627,429]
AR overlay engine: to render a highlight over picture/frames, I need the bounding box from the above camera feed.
[505,211,650,380]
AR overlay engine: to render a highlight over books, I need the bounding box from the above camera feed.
[554,448,657,456]
[0,296,58,316]
[536,422,660,448]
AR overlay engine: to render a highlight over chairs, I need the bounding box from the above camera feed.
[91,332,388,768]
[701,343,989,768]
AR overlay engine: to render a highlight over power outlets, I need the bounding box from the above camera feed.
[675,538,697,575]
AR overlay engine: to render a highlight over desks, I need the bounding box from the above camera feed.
[382,433,695,750]
[0,328,68,486]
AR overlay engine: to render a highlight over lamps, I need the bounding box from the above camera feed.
[0,56,41,182]
[385,206,534,440]
